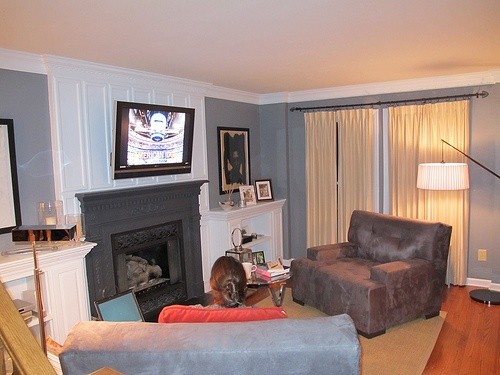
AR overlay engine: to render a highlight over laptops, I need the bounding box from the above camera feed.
[94,288,146,322]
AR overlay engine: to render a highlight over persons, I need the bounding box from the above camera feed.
[190,256,257,309]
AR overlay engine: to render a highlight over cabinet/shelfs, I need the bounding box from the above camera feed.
[0,229,97,362]
[200,198,287,294]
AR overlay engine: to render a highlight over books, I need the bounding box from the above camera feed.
[13,298,36,325]
[257,262,291,277]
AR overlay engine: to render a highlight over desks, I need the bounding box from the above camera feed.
[244,263,293,307]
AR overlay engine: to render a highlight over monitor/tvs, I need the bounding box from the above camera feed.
[113,100,196,180]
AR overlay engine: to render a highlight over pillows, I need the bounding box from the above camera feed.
[159,306,289,328]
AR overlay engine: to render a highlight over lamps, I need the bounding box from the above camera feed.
[416,140,500,190]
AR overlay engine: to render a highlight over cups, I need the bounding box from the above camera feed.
[242,263,257,279]
[65,212,86,242]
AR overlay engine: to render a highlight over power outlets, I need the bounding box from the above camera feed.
[477,250,487,263]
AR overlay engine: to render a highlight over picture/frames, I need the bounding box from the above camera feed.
[238,186,257,208]
[256,180,274,201]
[218,128,252,194]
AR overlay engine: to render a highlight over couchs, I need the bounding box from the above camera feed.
[58,313,362,374]
[291,211,453,339]
